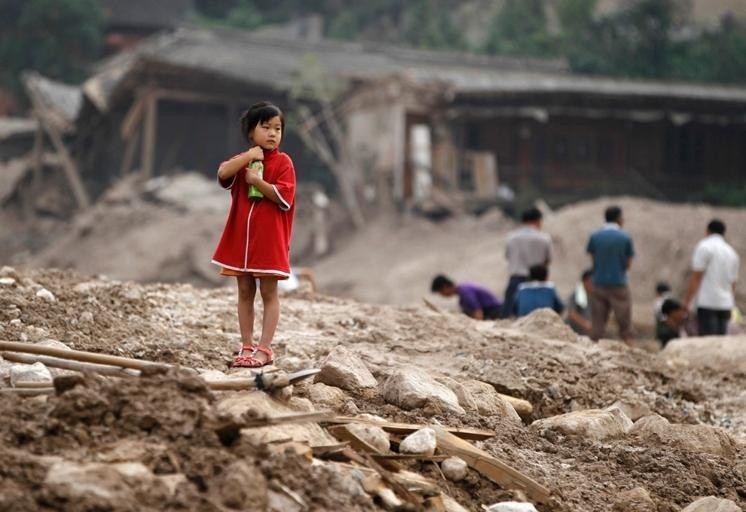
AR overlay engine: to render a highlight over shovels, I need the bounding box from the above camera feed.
[0,341,321,391]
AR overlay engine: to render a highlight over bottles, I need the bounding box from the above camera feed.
[246,159,264,201]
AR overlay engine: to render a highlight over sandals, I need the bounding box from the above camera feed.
[235,346,253,368]
[243,346,274,368]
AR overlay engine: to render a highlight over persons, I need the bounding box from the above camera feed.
[567,268,606,336]
[513,262,565,317]
[669,218,740,335]
[586,205,637,348]
[654,281,672,311]
[655,298,686,344]
[431,270,502,321]
[503,207,554,315]
[216,99,298,368]
